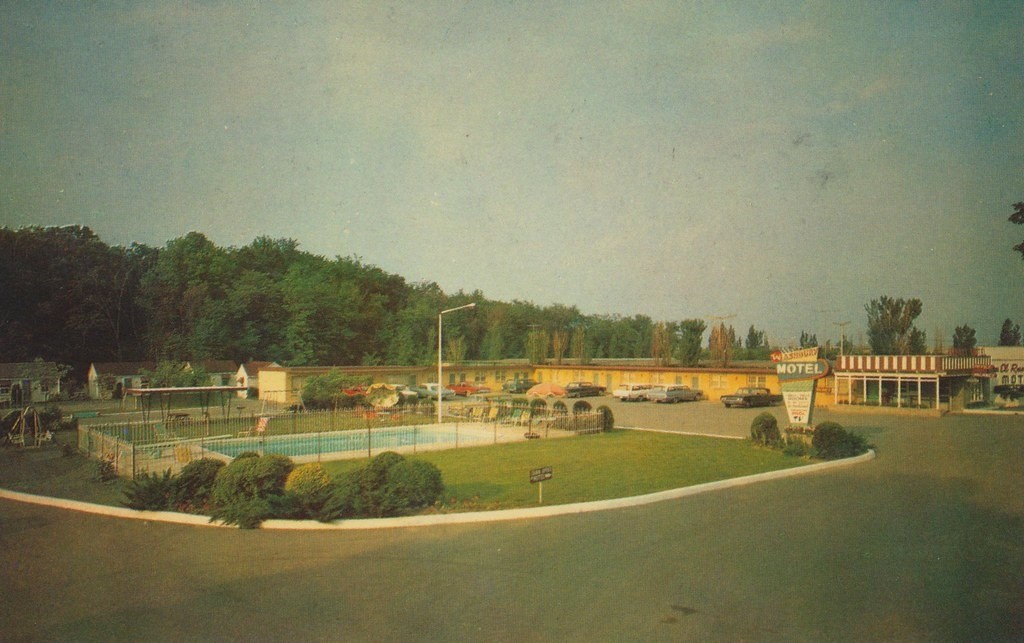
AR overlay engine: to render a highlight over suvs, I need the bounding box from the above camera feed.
[649,384,703,402]
[391,384,419,407]
[612,383,654,401]
[500,378,541,395]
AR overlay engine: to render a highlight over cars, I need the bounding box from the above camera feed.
[526,382,569,400]
[720,387,783,407]
[564,382,607,398]
[445,382,491,396]
[410,383,456,400]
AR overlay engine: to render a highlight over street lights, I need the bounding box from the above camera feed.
[436,302,477,423]
[833,320,851,356]
